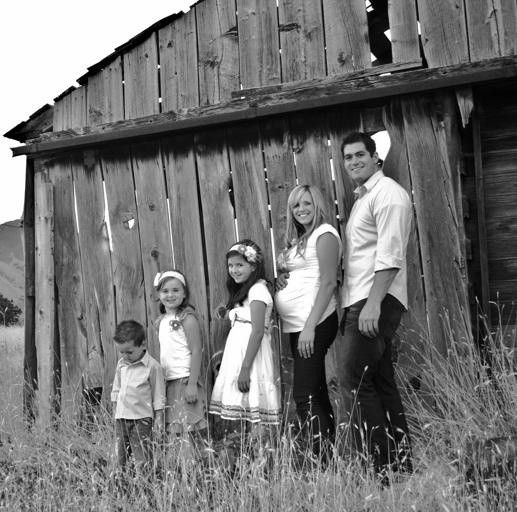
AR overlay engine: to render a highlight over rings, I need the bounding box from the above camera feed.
[305,344,309,347]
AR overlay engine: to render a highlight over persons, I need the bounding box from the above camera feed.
[152,270,210,442]
[108,319,168,482]
[272,185,342,476]
[333,132,412,489]
[207,239,282,446]
